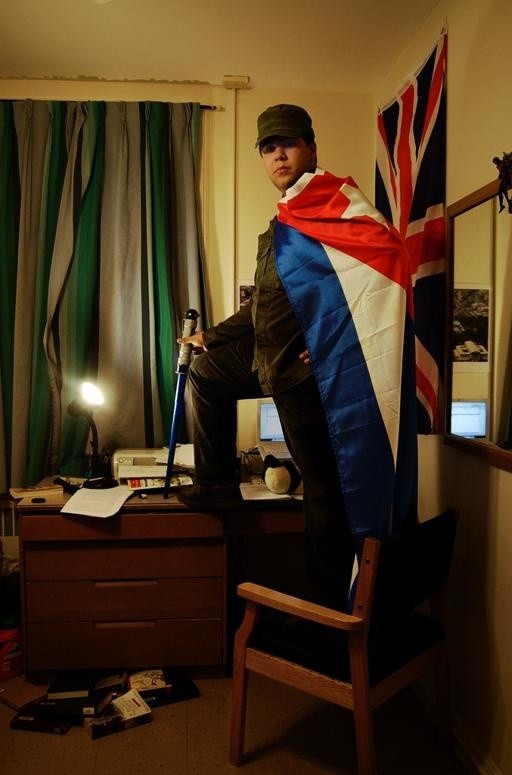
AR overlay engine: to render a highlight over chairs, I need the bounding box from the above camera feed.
[229,509,458,775]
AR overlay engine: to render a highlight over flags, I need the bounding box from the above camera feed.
[374,29,448,434]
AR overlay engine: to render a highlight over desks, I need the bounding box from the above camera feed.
[17,475,306,673]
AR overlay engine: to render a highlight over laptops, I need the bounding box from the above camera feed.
[449,399,489,446]
[256,398,292,462]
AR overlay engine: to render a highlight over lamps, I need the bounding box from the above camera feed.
[68,380,113,476]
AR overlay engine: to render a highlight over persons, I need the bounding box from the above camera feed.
[173,103,420,628]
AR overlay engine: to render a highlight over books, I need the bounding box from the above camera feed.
[2,668,174,738]
[9,483,65,498]
[117,464,168,479]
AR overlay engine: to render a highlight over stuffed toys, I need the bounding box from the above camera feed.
[261,453,302,493]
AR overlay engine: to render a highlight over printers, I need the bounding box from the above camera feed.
[114,447,178,479]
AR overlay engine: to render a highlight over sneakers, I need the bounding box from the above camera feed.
[177,479,242,509]
[283,616,322,640]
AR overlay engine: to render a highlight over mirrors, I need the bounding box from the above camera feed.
[444,175,512,473]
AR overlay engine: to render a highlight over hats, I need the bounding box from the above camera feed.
[255,104,310,147]
